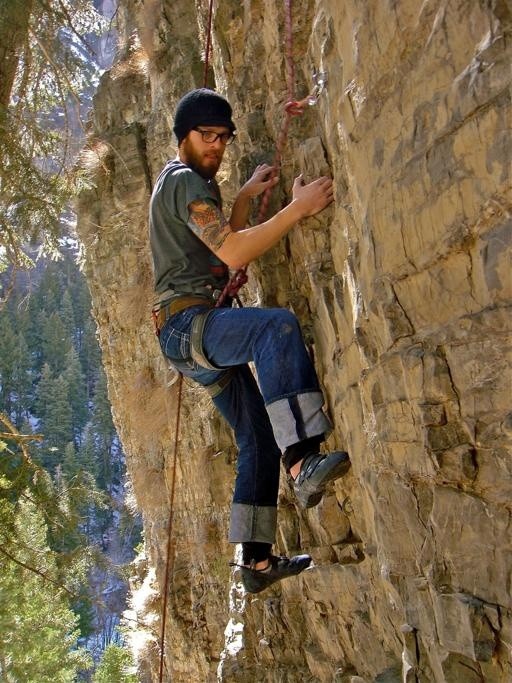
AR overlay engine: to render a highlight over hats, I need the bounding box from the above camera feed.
[173,88,236,148]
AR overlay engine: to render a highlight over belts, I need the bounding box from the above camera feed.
[157,297,208,328]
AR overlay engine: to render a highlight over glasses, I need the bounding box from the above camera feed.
[192,128,236,145]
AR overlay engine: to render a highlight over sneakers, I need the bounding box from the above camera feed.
[294,452,351,508]
[229,554,311,593]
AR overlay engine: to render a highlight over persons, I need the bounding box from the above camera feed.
[148,89,352,594]
